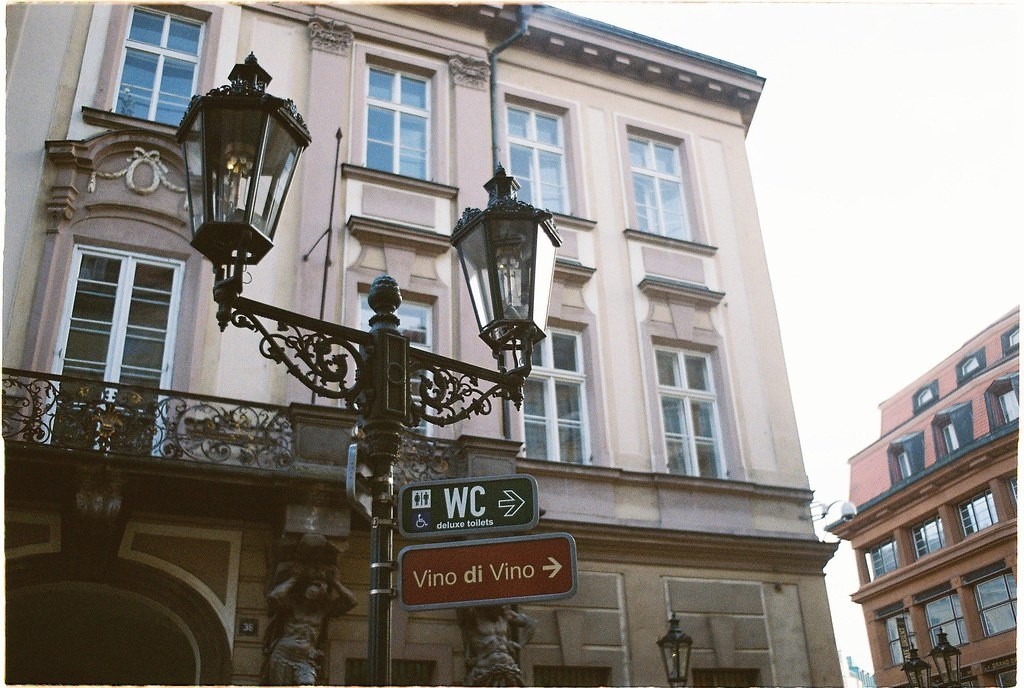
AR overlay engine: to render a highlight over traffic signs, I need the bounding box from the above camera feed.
[396,474,538,537]
[397,531,577,611]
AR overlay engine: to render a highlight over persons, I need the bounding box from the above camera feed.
[462,603,535,687]
[266,558,358,686]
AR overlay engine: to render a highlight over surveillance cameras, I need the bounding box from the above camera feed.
[841,502,857,521]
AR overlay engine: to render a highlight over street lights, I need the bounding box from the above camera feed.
[169,48,561,686]
